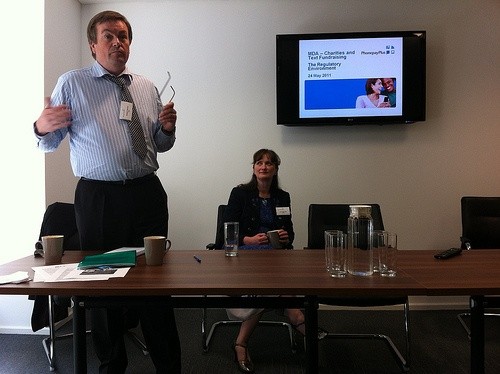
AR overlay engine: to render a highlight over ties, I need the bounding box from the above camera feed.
[102,74,148,160]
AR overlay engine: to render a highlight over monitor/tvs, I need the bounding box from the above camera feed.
[276,30,427,127]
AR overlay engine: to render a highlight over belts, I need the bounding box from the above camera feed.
[80,176,153,186]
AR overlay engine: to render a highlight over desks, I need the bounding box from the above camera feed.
[0,250,500,374]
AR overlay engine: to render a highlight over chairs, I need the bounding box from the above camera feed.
[457,197,500,340]
[202,205,298,355]
[29,202,149,372]
[303,203,411,374]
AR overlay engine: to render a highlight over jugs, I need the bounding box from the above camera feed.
[345,204,374,276]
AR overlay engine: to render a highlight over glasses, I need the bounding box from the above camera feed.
[152,72,175,115]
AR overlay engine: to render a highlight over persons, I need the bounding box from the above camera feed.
[356,77,396,108]
[228,148,327,372]
[33,11,184,374]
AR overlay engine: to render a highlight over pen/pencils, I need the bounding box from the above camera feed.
[193,255,201,262]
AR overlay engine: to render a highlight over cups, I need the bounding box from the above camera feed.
[374,230,399,279]
[144,236,171,265]
[266,230,281,249]
[325,230,347,278]
[223,222,239,257]
[35,234,63,266]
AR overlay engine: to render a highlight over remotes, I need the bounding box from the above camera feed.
[434,248,462,259]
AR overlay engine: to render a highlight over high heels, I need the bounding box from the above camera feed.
[232,336,256,373]
[289,319,329,341]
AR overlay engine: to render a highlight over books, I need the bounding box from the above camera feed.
[105,247,145,257]
